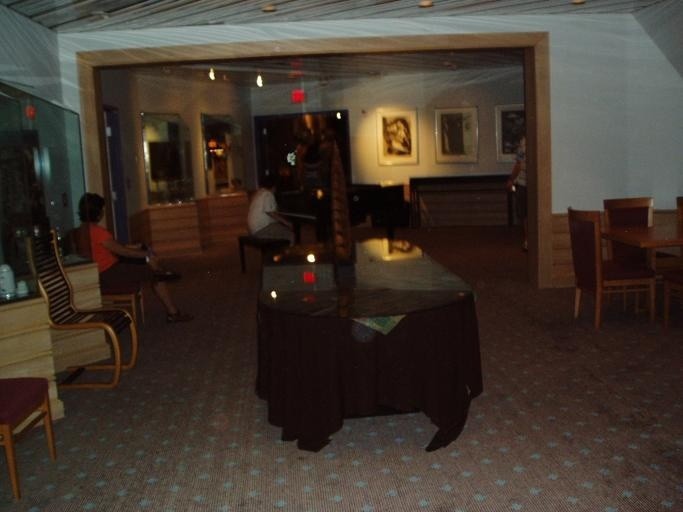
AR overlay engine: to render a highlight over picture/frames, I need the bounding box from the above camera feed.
[376,106,419,167]
[494,104,526,162]
[433,107,480,164]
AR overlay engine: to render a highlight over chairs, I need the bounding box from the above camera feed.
[61,228,146,325]
[25,229,139,390]
[676,195,682,208]
[0,377,57,501]
[568,206,656,333]
[602,197,676,311]
[661,270,682,329]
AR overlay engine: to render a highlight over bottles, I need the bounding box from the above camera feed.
[0,263,16,299]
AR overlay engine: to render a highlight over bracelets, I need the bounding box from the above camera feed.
[145,251,152,262]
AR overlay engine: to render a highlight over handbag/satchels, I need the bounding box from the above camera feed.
[142,242,185,284]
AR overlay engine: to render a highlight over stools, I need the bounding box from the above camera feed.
[238,234,290,273]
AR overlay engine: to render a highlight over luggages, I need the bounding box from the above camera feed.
[166,305,182,324]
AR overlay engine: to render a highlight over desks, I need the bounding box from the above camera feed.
[600,220,683,325]
[408,174,519,229]
[254,241,483,451]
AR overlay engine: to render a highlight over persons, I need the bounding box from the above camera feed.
[62,192,195,323]
[247,177,297,249]
[503,136,529,250]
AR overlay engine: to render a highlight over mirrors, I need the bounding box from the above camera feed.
[199,111,245,196]
[0,82,87,278]
[140,111,196,205]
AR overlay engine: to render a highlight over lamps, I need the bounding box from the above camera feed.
[24,96,37,121]
